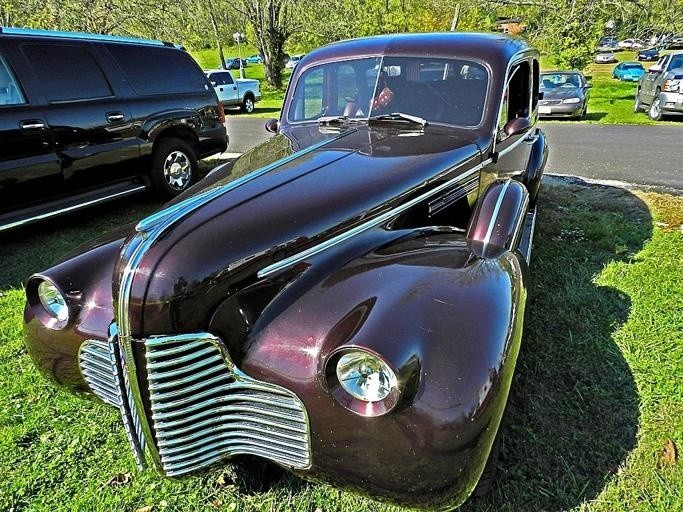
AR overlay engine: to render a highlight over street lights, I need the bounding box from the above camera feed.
[231,32,246,79]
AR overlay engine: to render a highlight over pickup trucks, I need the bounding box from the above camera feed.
[201,69,261,113]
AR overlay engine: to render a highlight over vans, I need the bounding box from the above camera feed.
[0,26,228,231]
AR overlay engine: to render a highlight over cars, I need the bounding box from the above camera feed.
[244,53,263,63]
[280,53,304,69]
[600,34,682,52]
[611,62,644,82]
[22,33,547,512]
[595,52,613,63]
[217,58,246,70]
[637,49,659,61]
[537,69,592,119]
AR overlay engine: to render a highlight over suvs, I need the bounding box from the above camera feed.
[632,53,683,120]
